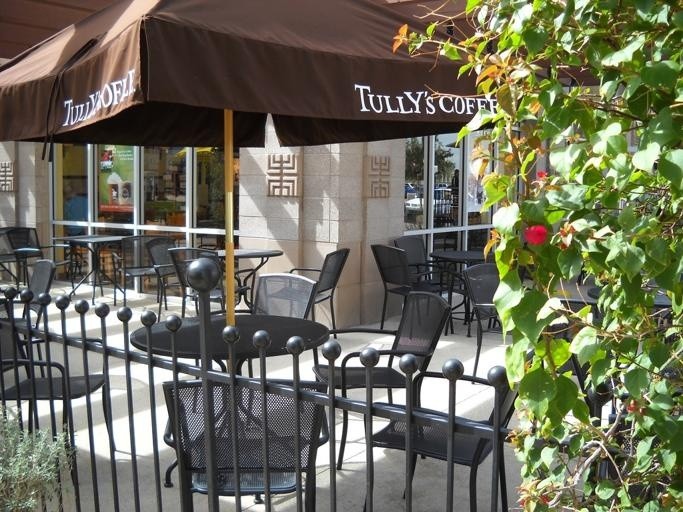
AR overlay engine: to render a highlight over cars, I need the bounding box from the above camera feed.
[404,182,453,218]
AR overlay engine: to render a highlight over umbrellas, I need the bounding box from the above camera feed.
[0,0,547,325]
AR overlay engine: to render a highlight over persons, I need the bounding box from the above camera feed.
[65,192,88,248]
[111,187,117,200]
[451,168,459,194]
[102,151,108,160]
[122,186,129,200]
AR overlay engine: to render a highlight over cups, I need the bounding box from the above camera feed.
[118,181,133,206]
[108,184,121,206]
